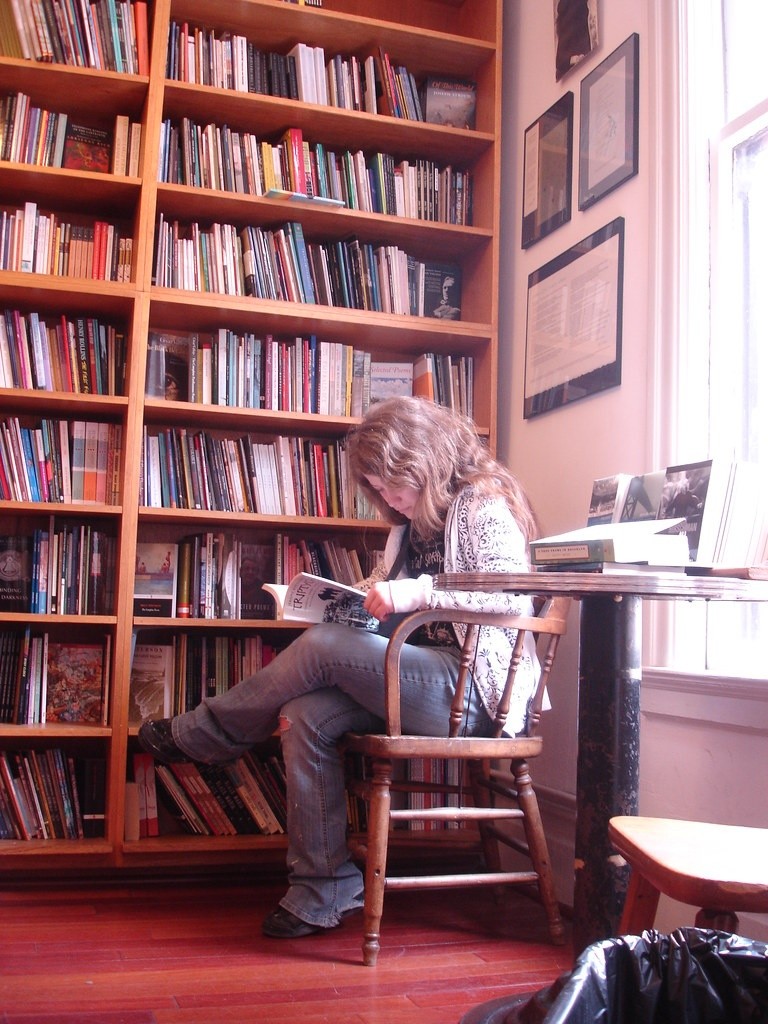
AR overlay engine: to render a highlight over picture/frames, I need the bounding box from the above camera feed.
[522,91,574,249]
[521,216,623,419]
[578,33,638,210]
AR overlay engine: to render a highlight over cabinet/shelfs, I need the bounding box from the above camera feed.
[0,0,505,880]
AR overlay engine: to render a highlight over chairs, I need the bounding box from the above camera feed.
[607,815,767,947]
[344,596,571,967]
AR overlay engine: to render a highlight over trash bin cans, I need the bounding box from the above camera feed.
[543,925,768,1024]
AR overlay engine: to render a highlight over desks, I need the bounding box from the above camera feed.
[431,568,767,1023]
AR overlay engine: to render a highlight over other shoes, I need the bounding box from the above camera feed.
[137,718,199,765]
[261,891,364,939]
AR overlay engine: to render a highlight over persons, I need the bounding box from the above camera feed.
[665,482,703,518]
[136,396,551,939]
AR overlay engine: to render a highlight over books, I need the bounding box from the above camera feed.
[0,3,477,841]
[261,572,380,632]
[530,458,768,581]
